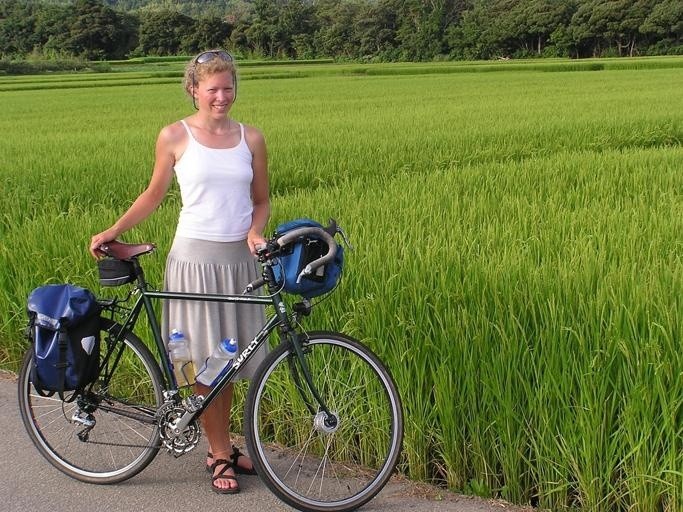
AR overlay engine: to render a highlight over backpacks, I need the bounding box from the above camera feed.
[24,283,101,403]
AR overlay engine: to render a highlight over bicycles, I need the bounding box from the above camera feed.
[18,216,405,511]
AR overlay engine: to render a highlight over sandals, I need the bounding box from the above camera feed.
[206,444,258,494]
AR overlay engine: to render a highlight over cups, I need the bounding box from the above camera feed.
[197,338,237,387]
[167,329,195,388]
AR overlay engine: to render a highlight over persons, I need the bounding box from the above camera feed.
[90,48,270,495]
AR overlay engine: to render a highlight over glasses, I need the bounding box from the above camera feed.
[193,51,232,65]
